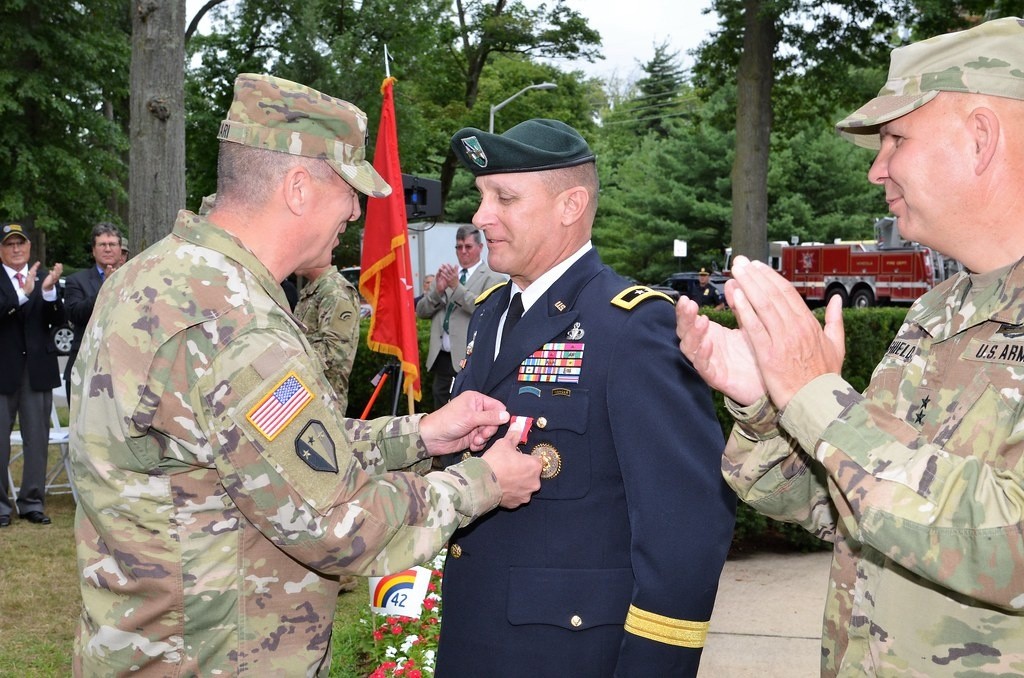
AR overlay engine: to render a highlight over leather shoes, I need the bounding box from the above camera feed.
[20,511,52,524]
[0,515,12,526]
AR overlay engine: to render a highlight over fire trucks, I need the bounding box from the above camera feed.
[722,216,965,309]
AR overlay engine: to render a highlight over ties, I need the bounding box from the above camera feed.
[443,269,468,335]
[14,273,25,288]
[500,292,524,349]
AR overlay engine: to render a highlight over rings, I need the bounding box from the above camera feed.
[28,272,30,275]
[448,274,449,276]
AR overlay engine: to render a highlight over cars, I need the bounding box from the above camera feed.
[340,266,373,320]
[644,285,678,304]
[28,275,75,355]
[657,271,730,309]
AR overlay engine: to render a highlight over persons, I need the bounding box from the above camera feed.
[120,237,129,265]
[62,223,122,410]
[72,73,546,678]
[416,226,509,409]
[692,267,725,311]
[414,273,435,312]
[293,267,360,417]
[434,118,736,678]
[679,13,1024,678]
[0,223,63,526]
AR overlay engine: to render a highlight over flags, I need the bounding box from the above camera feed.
[358,76,422,402]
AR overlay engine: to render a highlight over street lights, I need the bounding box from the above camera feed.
[489,83,558,135]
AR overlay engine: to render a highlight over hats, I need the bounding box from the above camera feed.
[0,222,30,244]
[200,193,217,217]
[218,73,393,198]
[121,237,130,253]
[836,16,1024,150]
[698,267,712,276]
[451,118,595,176]
